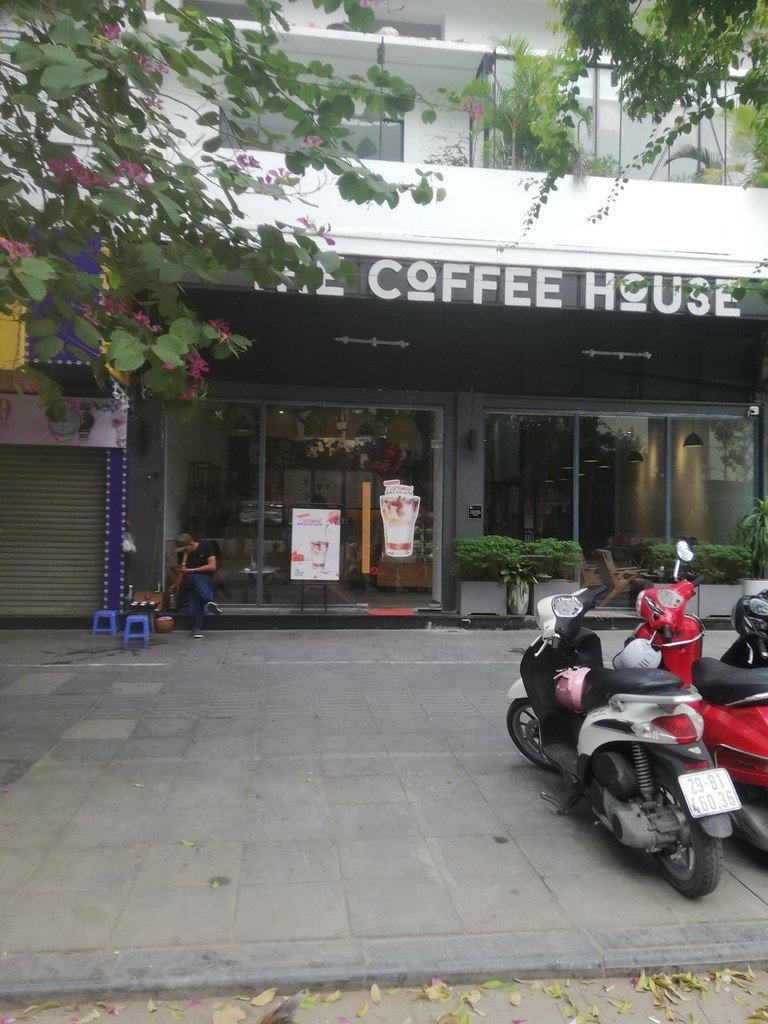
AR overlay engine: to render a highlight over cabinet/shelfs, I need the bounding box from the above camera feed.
[376,517,434,592]
[185,462,221,537]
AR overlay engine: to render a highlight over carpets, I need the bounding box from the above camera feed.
[368,609,415,616]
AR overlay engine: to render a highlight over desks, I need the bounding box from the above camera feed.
[642,573,674,583]
[237,565,280,605]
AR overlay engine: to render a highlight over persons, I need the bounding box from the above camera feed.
[175,533,222,638]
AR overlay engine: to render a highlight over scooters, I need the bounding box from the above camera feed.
[621,536,768,856]
[720,588,768,671]
[503,583,745,901]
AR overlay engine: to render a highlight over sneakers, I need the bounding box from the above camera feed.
[192,626,204,638]
[207,602,223,616]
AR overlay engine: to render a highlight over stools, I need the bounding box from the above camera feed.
[128,609,156,634]
[188,590,213,615]
[124,614,150,644]
[92,610,120,635]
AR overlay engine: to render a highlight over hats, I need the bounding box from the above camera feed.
[173,533,192,552]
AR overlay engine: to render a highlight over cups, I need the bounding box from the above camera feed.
[309,540,329,568]
[379,496,421,557]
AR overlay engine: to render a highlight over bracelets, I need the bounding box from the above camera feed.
[181,560,186,564]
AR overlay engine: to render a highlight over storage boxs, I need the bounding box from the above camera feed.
[133,591,165,610]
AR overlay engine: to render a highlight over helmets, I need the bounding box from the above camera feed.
[552,666,591,714]
[612,638,662,676]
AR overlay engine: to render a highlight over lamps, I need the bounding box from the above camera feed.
[468,429,477,451]
[626,450,643,463]
[141,420,151,448]
[682,420,704,448]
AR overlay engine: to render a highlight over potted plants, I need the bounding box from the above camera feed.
[645,496,768,618]
[451,537,582,614]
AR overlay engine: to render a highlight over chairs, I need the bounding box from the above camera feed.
[580,548,652,607]
[294,544,361,603]
[165,537,234,601]
